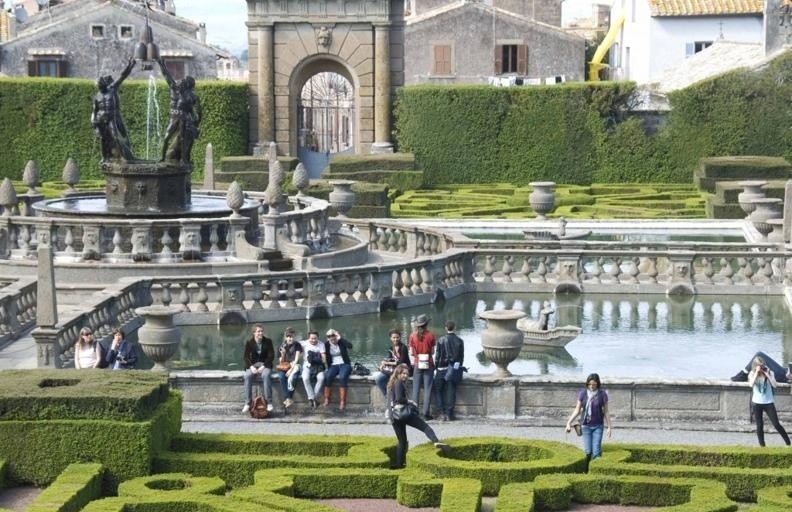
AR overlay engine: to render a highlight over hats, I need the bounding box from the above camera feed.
[415,314,429,327]
[285,327,296,337]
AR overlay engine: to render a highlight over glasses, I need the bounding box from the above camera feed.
[327,334,336,338]
[83,332,92,336]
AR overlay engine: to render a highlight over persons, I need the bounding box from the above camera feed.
[320,329,352,412]
[75,327,107,369]
[749,356,790,446]
[565,373,611,459]
[730,351,792,385]
[432,321,464,421]
[241,323,274,412]
[90,56,202,165]
[387,363,448,468]
[374,330,412,399]
[408,314,436,419]
[295,331,328,411]
[104,328,138,370]
[279,328,302,408]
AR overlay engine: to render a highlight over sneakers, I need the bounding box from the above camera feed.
[266,404,273,411]
[282,397,294,408]
[241,405,250,412]
[385,409,391,419]
[423,415,433,421]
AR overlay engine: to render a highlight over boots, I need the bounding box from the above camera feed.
[322,386,331,407]
[338,387,348,410]
[309,398,320,412]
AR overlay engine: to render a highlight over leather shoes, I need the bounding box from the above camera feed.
[439,415,456,421]
[434,442,450,448]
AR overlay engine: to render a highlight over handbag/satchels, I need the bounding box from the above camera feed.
[444,362,468,383]
[93,340,110,369]
[390,401,416,423]
[250,396,272,419]
[573,422,585,436]
[377,359,394,376]
[352,362,372,375]
[307,350,323,366]
[276,361,292,371]
[417,353,431,370]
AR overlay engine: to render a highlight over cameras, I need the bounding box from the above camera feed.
[760,365,767,371]
